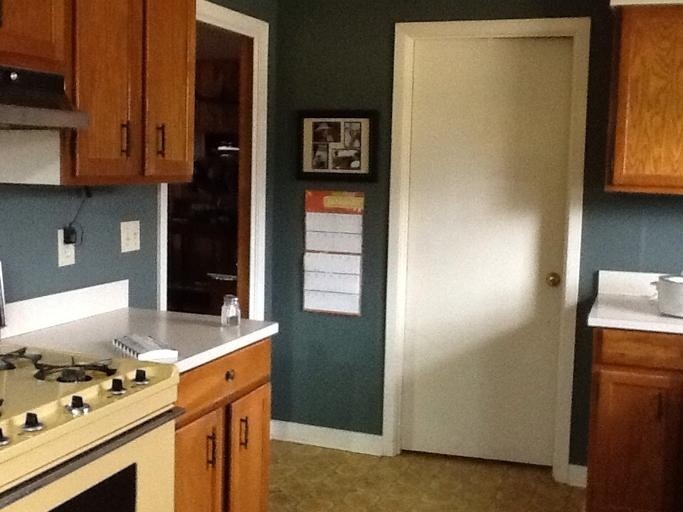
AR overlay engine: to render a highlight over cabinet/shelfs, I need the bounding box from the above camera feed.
[587,326,683,512]
[603,0,683,196]
[175,337,272,512]
[0,0,196,185]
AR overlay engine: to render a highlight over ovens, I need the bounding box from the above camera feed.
[0,405,188,512]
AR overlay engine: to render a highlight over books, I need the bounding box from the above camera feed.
[112,330,178,362]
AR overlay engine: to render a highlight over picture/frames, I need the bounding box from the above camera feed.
[296,110,378,183]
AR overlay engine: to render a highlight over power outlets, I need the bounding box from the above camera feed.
[121,221,141,253]
[58,230,76,267]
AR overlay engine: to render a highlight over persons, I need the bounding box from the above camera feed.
[313,122,335,142]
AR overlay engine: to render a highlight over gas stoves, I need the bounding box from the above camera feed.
[0,338,180,453]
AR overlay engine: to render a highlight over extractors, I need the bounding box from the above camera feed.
[0,67,90,130]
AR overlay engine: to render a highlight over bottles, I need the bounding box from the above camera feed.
[221,295,241,328]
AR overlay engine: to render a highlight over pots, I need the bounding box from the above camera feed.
[650,274,683,318]
